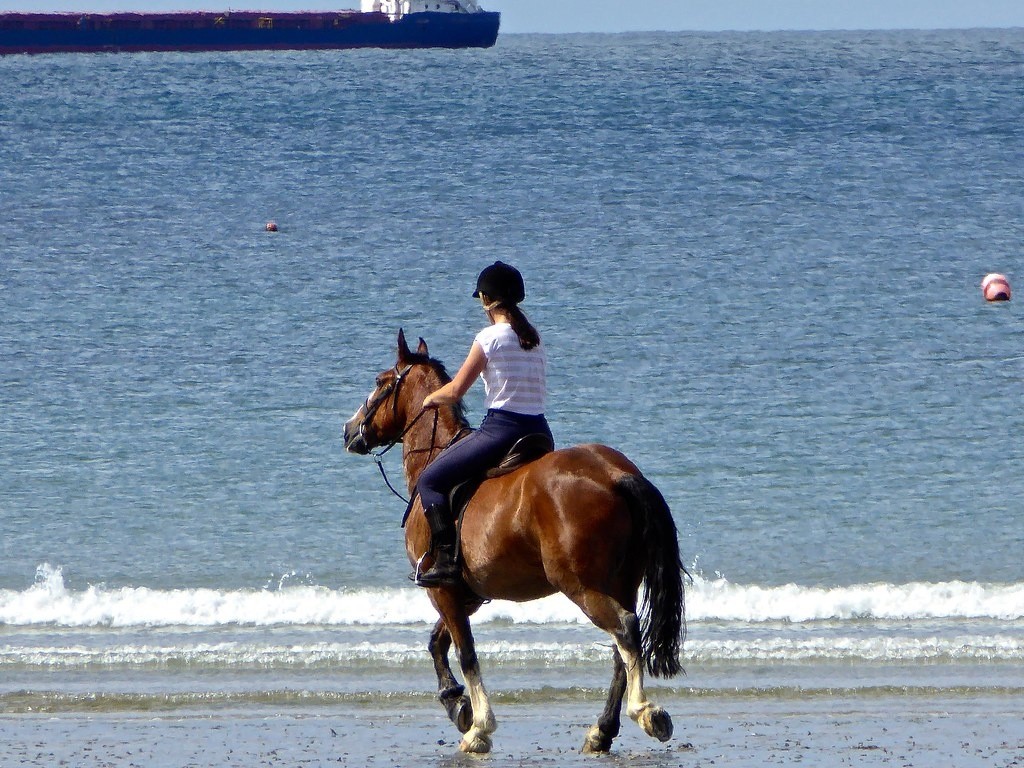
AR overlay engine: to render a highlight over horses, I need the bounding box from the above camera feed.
[343,328,694,759]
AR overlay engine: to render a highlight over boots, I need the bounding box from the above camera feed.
[408,503,463,586]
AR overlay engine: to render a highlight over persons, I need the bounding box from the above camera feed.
[407,260,554,590]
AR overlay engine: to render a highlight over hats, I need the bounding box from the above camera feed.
[473,261,524,302]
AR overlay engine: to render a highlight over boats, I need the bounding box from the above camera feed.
[0,0,499,56]
